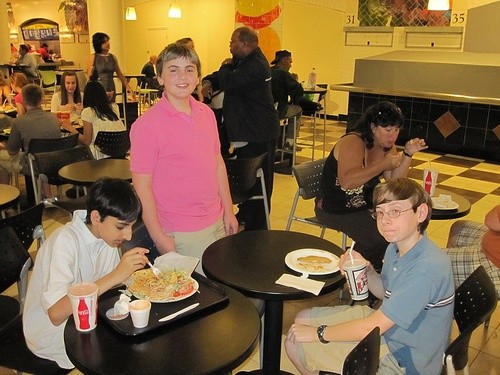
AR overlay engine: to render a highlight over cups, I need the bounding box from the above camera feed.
[344,258,370,301]
[129,300,151,328]
[423,168,439,197]
[67,282,99,333]
[8,94,15,106]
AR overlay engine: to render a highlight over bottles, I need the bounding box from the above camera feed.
[0,88,5,105]
[308,67,317,90]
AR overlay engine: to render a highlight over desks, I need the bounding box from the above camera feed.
[0,64,31,104]
[279,105,302,176]
[59,69,83,90]
[0,183,21,219]
[58,158,131,197]
[303,87,328,161]
[113,74,146,129]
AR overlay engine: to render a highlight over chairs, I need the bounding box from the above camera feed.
[0,59,499,375]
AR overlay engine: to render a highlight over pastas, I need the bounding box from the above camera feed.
[143,270,180,297]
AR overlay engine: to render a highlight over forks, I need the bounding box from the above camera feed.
[147,262,163,279]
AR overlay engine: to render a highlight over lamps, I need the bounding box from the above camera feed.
[125,0,136,20]
[168,0,181,18]
[428,0,450,10]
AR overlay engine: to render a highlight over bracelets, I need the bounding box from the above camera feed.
[317,325,330,343]
[403,149,412,157]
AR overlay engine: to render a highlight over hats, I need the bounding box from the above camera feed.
[270,50,291,65]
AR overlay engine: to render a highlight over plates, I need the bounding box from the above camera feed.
[431,196,459,210]
[285,248,340,275]
[126,275,199,304]
[106,308,129,321]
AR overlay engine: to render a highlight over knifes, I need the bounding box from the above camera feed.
[158,303,200,322]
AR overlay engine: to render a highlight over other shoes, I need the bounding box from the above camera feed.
[284,141,302,152]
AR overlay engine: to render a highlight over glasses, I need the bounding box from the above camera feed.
[371,108,401,122]
[370,206,413,220]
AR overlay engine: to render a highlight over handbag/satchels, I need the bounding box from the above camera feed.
[88,65,100,81]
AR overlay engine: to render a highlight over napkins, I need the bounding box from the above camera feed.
[152,251,200,277]
[274,273,325,296]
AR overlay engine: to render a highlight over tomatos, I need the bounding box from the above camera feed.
[173,283,194,297]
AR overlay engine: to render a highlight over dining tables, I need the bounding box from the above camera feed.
[0,131,21,187]
[202,229,347,375]
[64,281,262,375]
[365,186,471,220]
[60,124,83,137]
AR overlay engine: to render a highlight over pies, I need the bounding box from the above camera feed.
[297,256,332,262]
[296,263,323,271]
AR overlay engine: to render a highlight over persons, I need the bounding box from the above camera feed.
[129,44,238,278]
[442,205,500,300]
[201,27,280,231]
[141,56,160,106]
[21,177,150,370]
[0,32,131,208]
[177,38,200,101]
[270,50,303,151]
[284,177,454,375]
[314,101,429,307]
